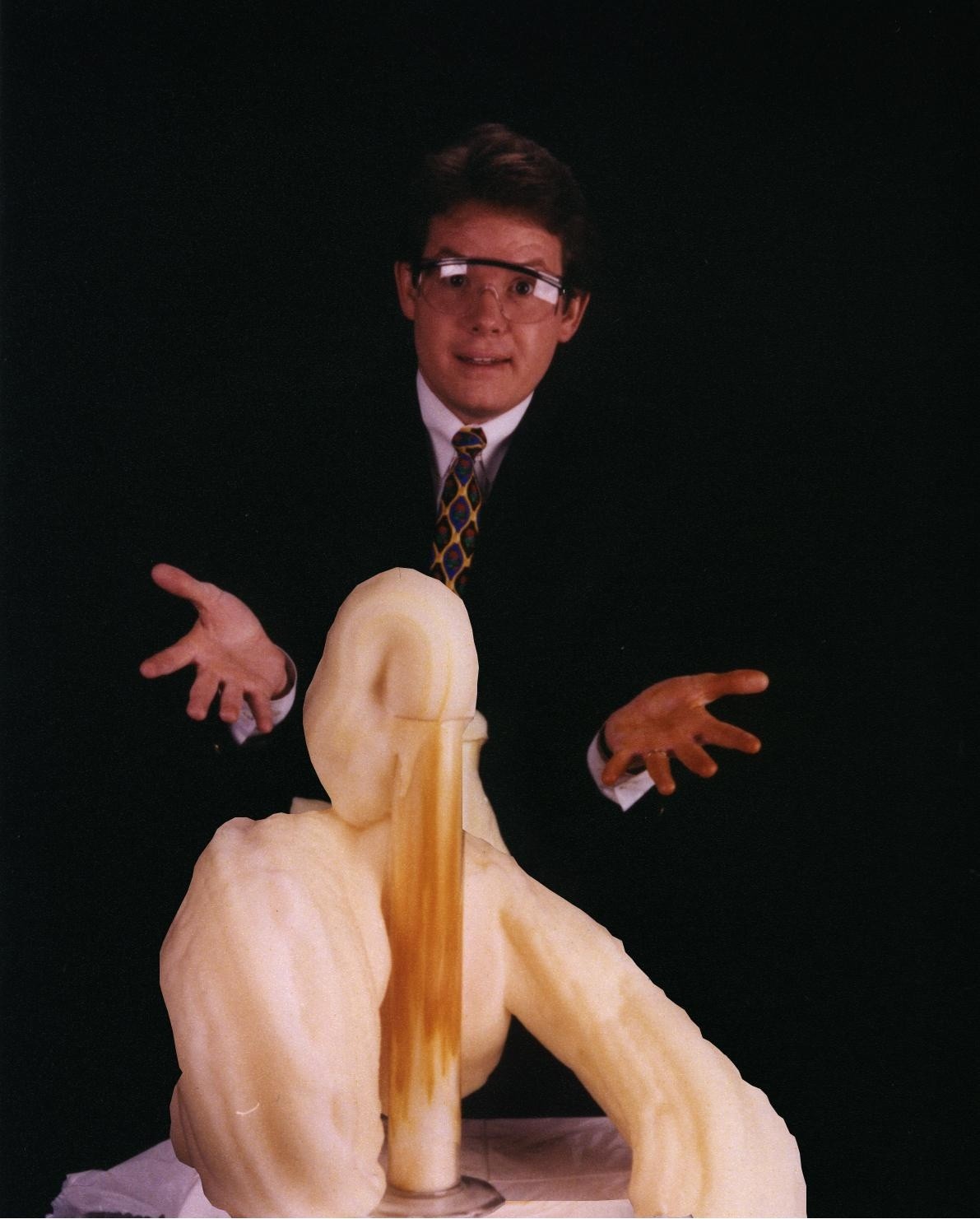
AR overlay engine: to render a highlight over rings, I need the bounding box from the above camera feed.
[645,749,667,757]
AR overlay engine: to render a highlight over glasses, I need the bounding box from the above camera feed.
[413,255,584,326]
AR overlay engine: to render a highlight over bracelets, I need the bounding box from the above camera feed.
[599,724,647,776]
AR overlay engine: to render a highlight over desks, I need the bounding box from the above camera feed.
[51,1117,685,1219]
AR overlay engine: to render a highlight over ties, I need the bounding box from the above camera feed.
[431,429,487,594]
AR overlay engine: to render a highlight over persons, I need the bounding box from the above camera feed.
[129,124,773,1124]
